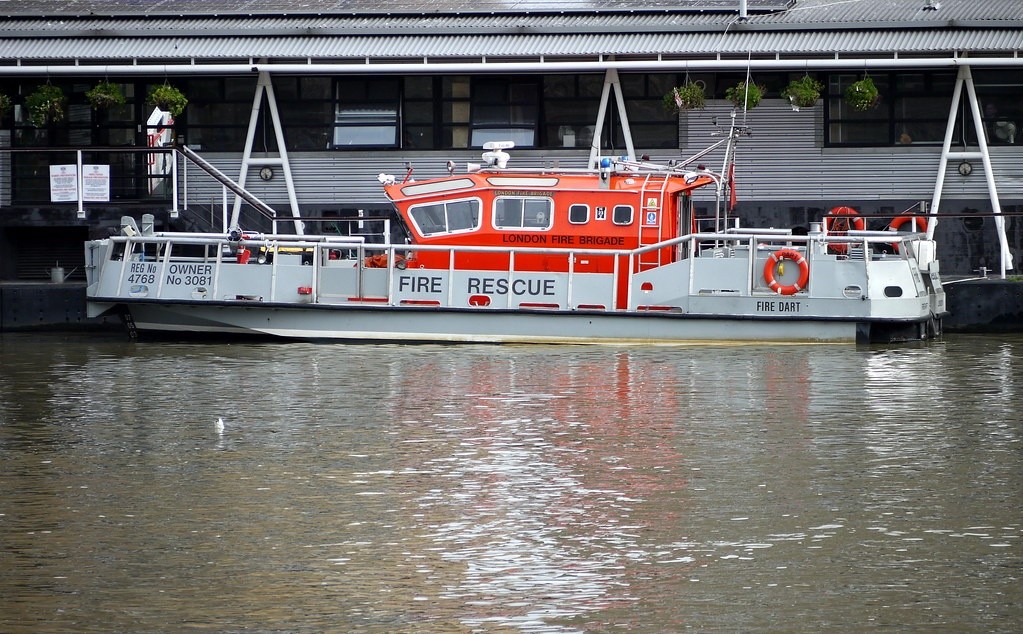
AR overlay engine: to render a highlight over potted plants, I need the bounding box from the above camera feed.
[145,86,191,117]
[844,78,879,113]
[661,82,707,115]
[24,84,65,128]
[781,74,825,112]
[85,83,126,127]
[726,82,766,112]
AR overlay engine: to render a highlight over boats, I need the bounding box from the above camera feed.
[76,52,1015,346]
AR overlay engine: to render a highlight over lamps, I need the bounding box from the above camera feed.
[397,260,406,270]
[684,173,697,184]
[257,253,266,264]
[447,161,456,177]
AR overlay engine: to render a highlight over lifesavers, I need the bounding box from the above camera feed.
[888,211,928,252]
[763,249,809,295]
[821,206,864,252]
